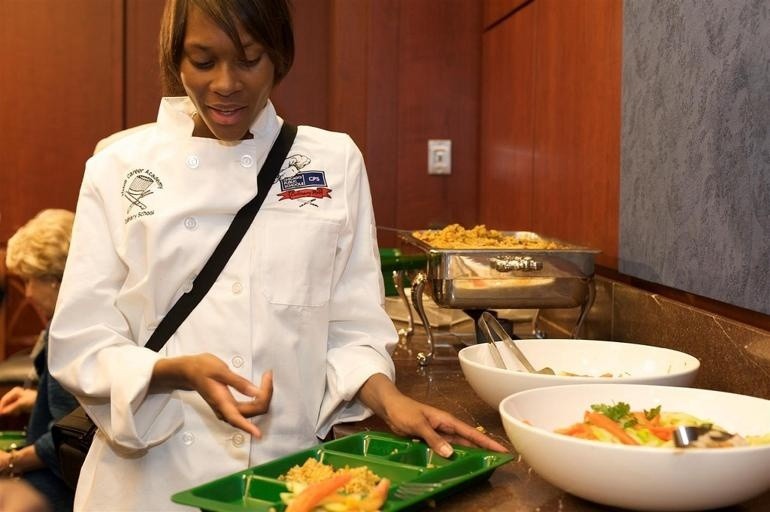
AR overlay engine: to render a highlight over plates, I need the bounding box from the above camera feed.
[171,430,515,512]
[0,430,27,451]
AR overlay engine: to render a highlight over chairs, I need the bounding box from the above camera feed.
[0,329,48,390]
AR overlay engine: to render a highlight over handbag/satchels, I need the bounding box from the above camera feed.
[50,120,297,493]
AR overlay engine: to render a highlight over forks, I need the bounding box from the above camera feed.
[393,476,465,501]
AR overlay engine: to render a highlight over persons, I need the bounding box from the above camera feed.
[0,210,98,511]
[49,0,508,512]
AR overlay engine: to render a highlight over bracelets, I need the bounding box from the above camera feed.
[5,445,20,478]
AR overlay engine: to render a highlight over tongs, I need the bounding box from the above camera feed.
[672,426,733,448]
[477,311,555,375]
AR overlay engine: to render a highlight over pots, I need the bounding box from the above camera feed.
[392,229,604,366]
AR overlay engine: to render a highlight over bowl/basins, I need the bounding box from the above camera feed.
[379,248,442,297]
[499,383,770,511]
[458,338,700,411]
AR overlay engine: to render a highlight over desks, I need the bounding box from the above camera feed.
[333,236,769,512]
